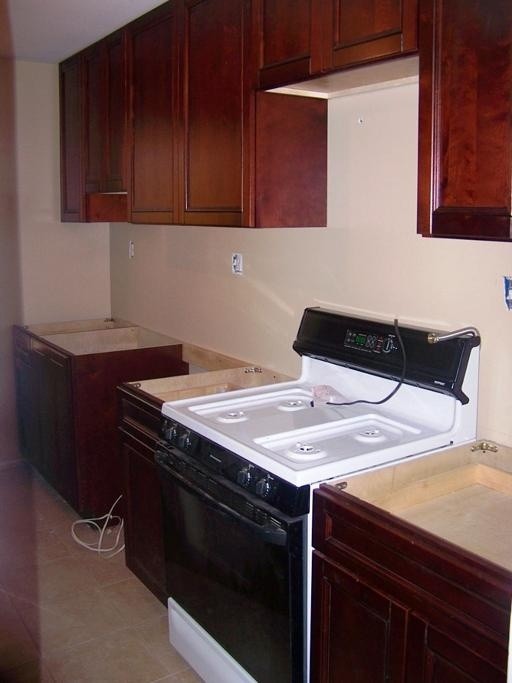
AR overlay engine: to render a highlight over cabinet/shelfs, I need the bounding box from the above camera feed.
[184,0,328,229]
[416,1,510,241]
[11,316,190,534]
[308,439,509,683]
[249,0,419,90]
[58,0,181,225]
[114,365,296,607]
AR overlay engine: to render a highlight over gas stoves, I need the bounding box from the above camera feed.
[159,354,459,517]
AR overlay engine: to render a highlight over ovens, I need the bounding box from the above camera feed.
[156,457,300,682]
